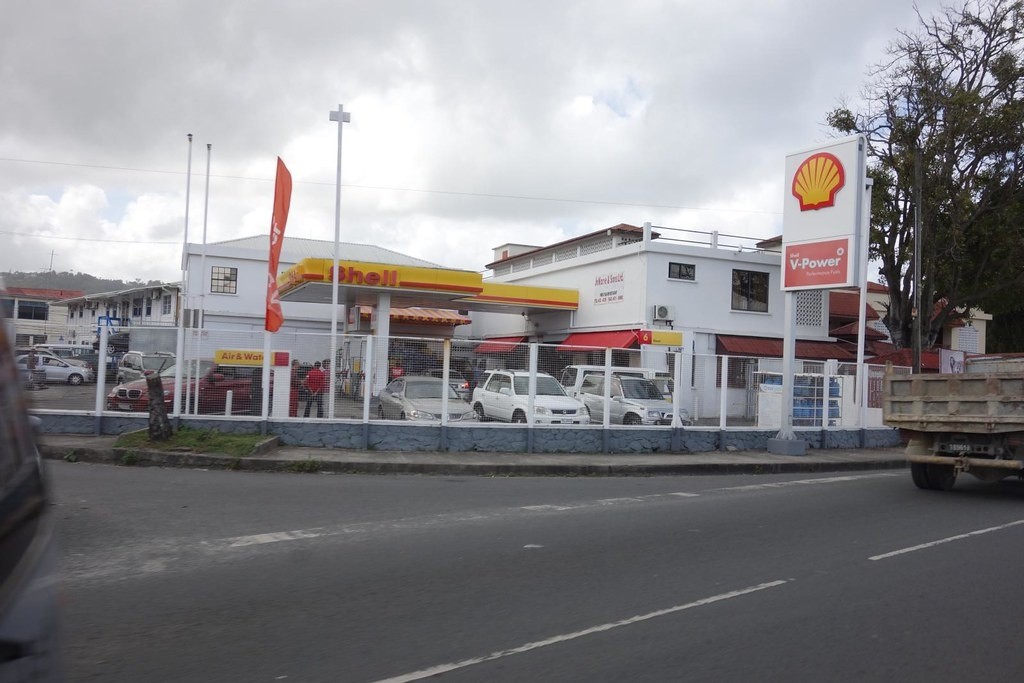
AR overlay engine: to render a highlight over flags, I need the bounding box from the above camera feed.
[265,157,293,332]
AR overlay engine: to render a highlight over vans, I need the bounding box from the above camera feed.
[560,364,674,410]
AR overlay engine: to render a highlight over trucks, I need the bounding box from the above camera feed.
[881,352,1024,492]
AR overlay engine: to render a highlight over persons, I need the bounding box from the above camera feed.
[26,349,36,369]
[304,361,325,418]
[322,359,330,418]
[289,359,304,417]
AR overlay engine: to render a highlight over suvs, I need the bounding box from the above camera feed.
[470,369,592,425]
[115,351,193,388]
[14,343,123,382]
[574,373,691,427]
[290,360,314,393]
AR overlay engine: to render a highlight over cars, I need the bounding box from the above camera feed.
[379,376,480,422]
[423,369,470,400]
[104,356,274,414]
[16,354,95,386]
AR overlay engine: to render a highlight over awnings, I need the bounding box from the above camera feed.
[357,305,472,327]
[554,330,639,352]
[471,336,527,353]
[717,334,858,362]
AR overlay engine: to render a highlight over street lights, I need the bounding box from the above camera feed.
[327,103,352,420]
